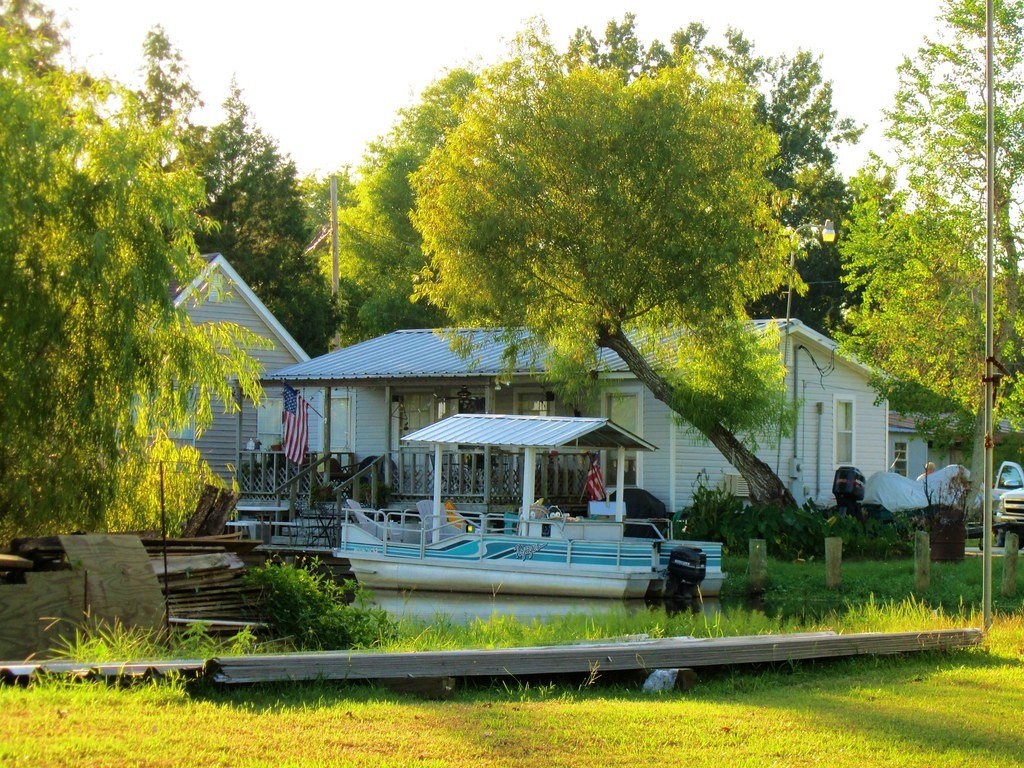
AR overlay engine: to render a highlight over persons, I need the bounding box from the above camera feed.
[947,465,968,496]
[993,494,1006,547]
[917,462,936,481]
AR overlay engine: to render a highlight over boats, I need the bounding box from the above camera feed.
[329,412,730,603]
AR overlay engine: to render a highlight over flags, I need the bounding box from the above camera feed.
[586,449,607,500]
[280,381,309,465]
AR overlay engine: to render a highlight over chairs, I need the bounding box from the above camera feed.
[296,456,383,501]
[293,500,337,549]
[346,499,392,540]
[417,500,463,534]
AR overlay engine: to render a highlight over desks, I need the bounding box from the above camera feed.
[521,517,624,542]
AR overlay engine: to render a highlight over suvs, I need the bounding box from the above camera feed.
[990,460,1024,550]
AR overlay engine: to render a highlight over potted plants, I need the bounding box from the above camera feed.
[270,435,282,451]
[315,479,341,501]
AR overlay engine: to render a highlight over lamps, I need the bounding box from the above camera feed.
[457,385,471,399]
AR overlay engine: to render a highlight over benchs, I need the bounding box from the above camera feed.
[227,520,301,547]
